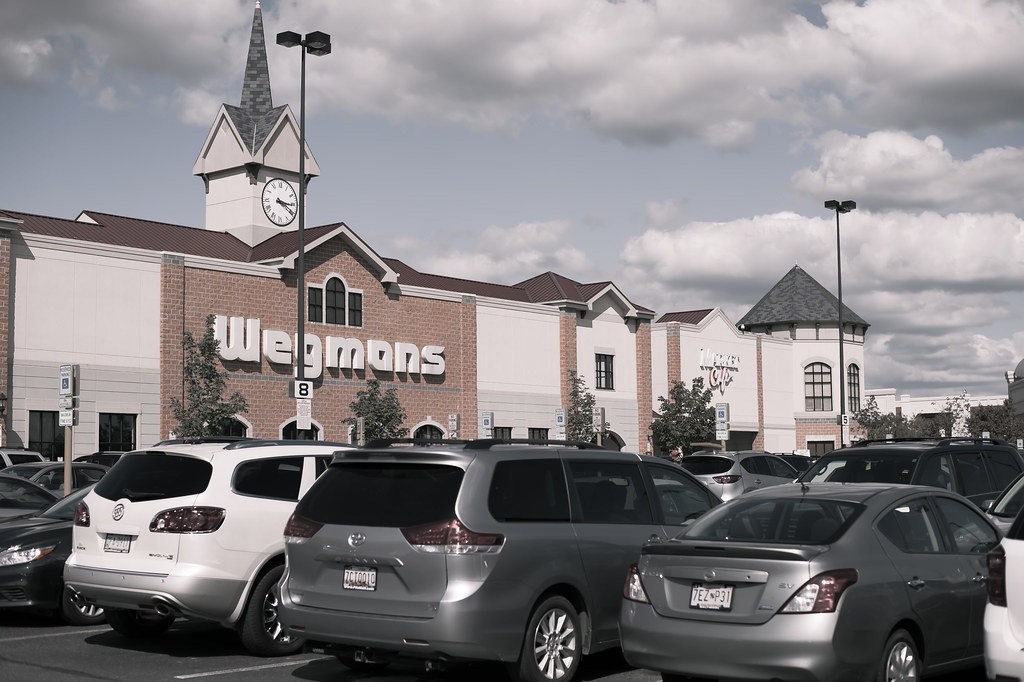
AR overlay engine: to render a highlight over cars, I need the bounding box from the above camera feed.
[0,473,61,522]
[619,479,1008,682]
[0,462,111,500]
[0,446,47,469]
[982,504,1023,682]
[1,480,115,626]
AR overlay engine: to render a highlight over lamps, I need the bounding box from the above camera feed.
[824,200,856,213]
[277,30,332,56]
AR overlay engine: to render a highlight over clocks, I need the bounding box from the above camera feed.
[261,178,298,226]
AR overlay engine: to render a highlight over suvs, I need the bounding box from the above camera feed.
[274,436,735,682]
[682,449,798,503]
[62,435,374,653]
[71,450,127,468]
[786,436,1024,524]
[770,453,813,476]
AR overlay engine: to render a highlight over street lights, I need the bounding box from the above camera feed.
[822,199,857,449]
[275,30,331,379]
